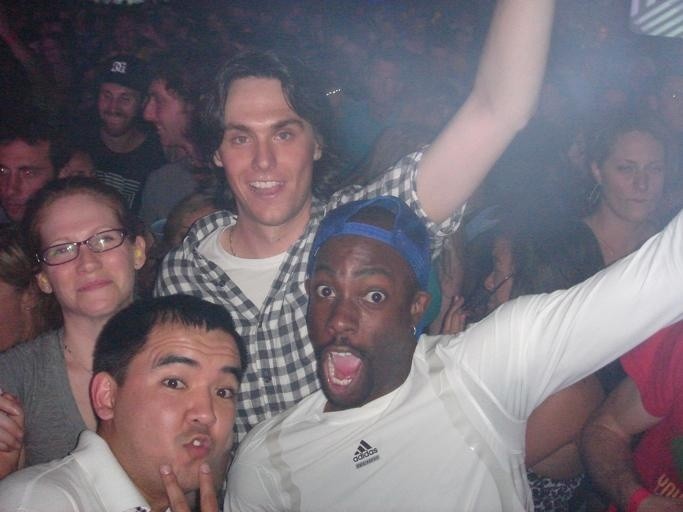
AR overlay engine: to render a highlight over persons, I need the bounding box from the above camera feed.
[2,2,682,512]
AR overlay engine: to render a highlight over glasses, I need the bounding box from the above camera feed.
[33,227,132,267]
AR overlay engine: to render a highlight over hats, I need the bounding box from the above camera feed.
[99,56,149,94]
[307,196,442,337]
